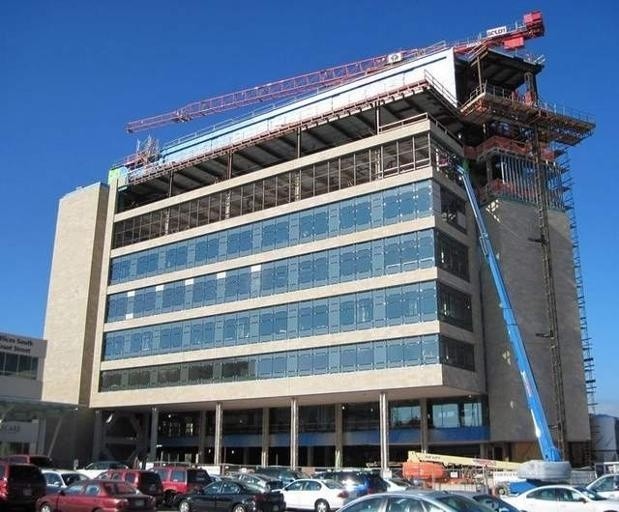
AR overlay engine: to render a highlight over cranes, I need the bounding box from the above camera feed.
[121,9,547,134]
